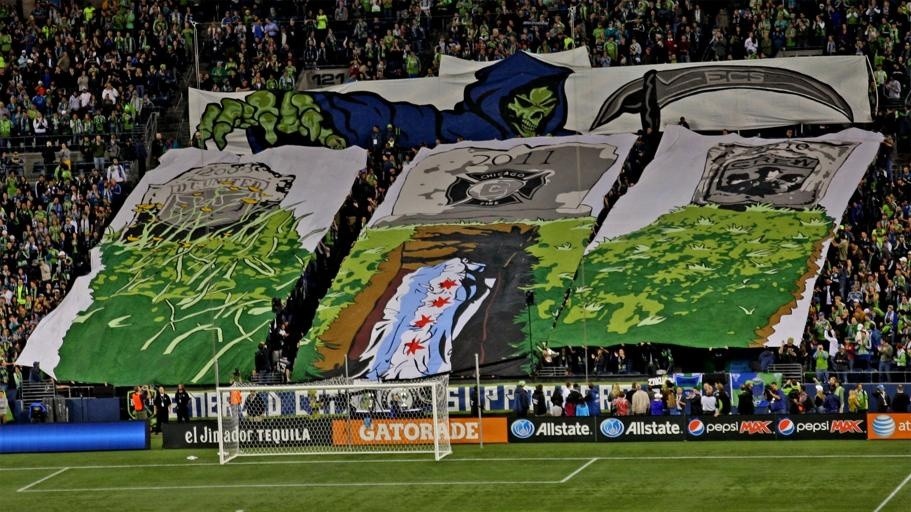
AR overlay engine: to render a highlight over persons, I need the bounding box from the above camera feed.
[2,1,911,432]
